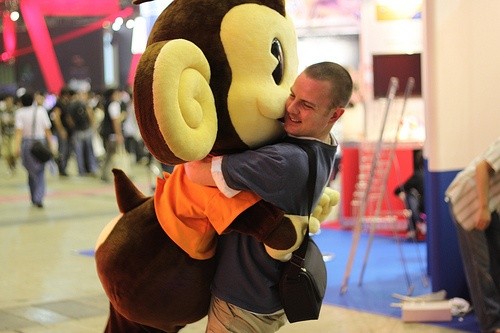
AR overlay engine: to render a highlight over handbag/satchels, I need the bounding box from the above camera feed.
[281,236,329,323]
[32,141,52,163]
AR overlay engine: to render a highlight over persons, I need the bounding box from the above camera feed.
[187,62,353,332]
[445,139,499,332]
[4,87,154,182]
[15,90,59,208]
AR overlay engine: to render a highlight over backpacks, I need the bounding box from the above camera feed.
[72,102,90,129]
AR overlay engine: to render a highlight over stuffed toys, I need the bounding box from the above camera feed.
[95,0,341,333]
